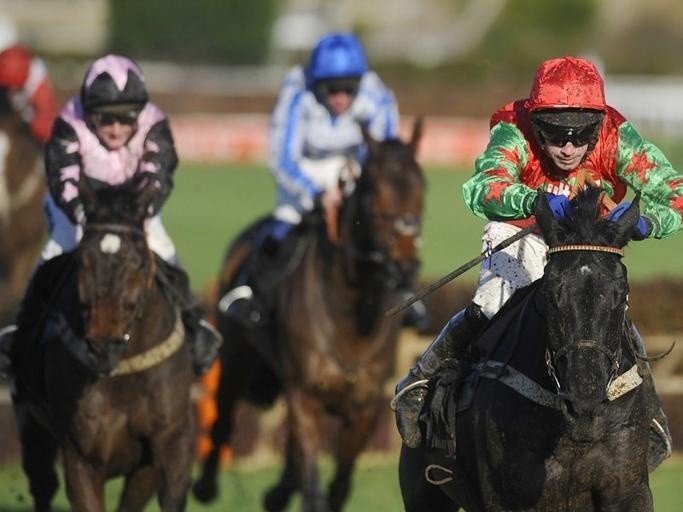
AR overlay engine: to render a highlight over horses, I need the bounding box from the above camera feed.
[0,84,51,470]
[9,172,199,512]
[396,183,660,512]
[191,115,429,512]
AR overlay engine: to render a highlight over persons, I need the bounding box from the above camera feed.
[392,55,683,475]
[0,46,59,144]
[0,52,225,378]
[225,31,400,328]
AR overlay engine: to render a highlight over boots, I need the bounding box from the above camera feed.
[395,301,488,451]
[627,319,673,471]
[220,216,298,333]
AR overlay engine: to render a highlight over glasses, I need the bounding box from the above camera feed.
[92,110,139,125]
[322,80,355,94]
[540,125,593,147]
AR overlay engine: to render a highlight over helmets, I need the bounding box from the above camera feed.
[531,108,603,151]
[80,53,150,109]
[309,33,366,81]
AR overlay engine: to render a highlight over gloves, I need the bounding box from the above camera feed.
[532,191,576,225]
[604,203,649,241]
